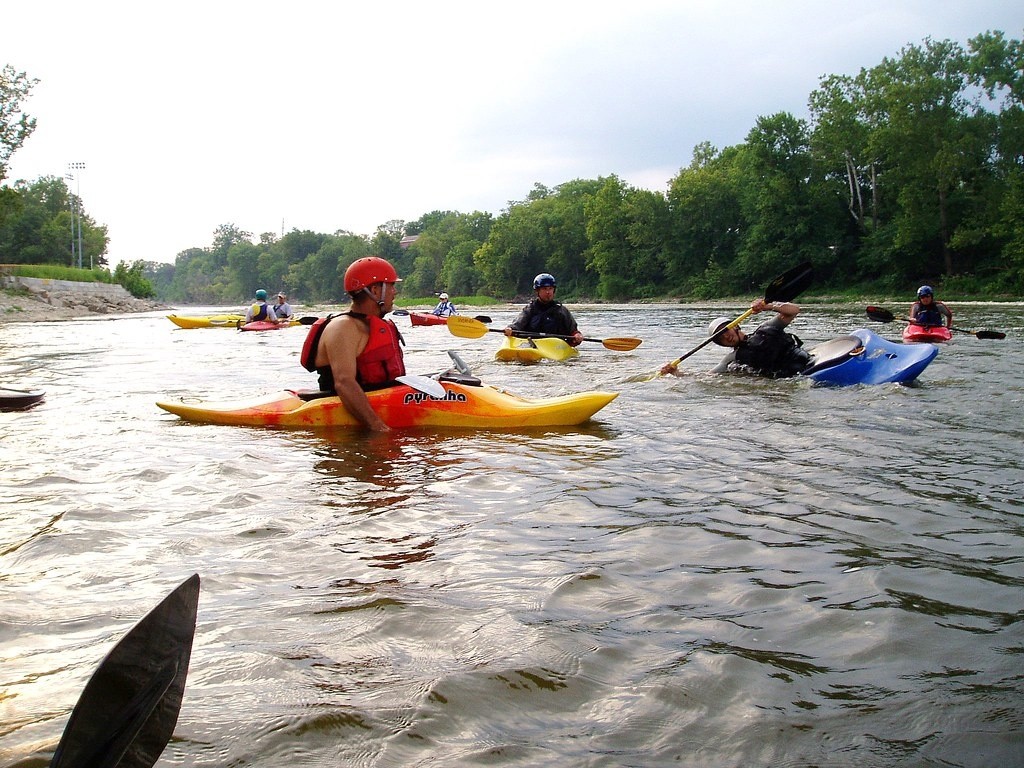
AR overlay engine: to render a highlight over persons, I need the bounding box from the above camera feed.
[909,286,952,329]
[300,257,405,432]
[246,289,294,324]
[661,299,810,378]
[433,293,461,318]
[504,273,583,347]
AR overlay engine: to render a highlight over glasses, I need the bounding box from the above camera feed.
[920,294,931,299]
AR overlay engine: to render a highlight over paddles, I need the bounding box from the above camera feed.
[236,319,240,330]
[656,261,815,375]
[446,314,644,353]
[866,305,1007,339]
[49,573,201,768]
[393,308,492,324]
[208,316,319,325]
[395,349,470,399]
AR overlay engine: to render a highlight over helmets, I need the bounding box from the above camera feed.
[532,273,557,289]
[344,257,403,291]
[708,317,732,343]
[916,285,934,301]
[439,293,448,299]
[256,289,267,300]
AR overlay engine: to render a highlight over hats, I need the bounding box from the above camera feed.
[278,294,287,299]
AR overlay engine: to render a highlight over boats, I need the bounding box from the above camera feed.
[494,334,582,363]
[0,384,46,408]
[239,321,290,331]
[153,370,621,429]
[409,312,447,327]
[166,313,302,330]
[901,324,953,341]
[798,328,940,390]
